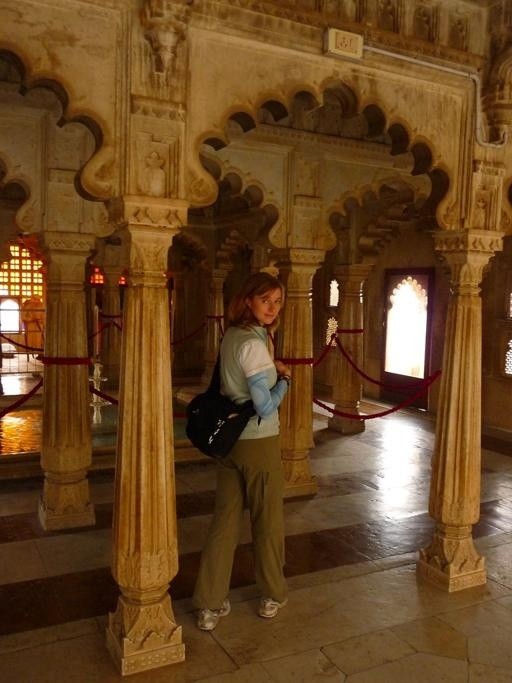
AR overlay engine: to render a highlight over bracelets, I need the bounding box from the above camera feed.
[281,374,292,385]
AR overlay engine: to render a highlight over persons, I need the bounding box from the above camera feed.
[189,268,294,632]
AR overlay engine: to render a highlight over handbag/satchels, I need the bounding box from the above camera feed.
[185,390,254,459]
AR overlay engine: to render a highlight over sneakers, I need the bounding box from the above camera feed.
[259,597,289,620]
[197,598,232,632]
